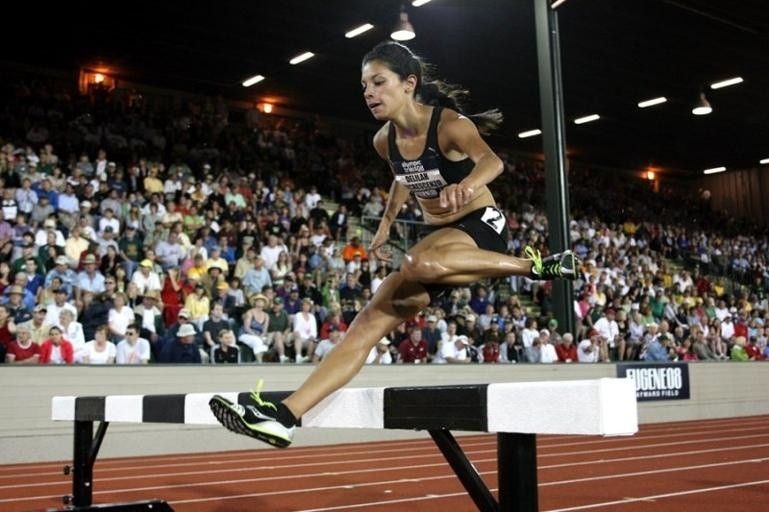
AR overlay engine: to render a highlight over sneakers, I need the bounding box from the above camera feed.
[209,379,296,448]
[525,246,579,280]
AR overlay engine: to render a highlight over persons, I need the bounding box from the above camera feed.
[211,40,580,448]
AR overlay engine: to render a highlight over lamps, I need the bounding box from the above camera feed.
[691,90,714,115]
[390,0,416,42]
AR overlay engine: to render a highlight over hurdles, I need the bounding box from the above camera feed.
[53,378,638,512]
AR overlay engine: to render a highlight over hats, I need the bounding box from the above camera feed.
[4,162,362,338]
[378,292,659,352]
